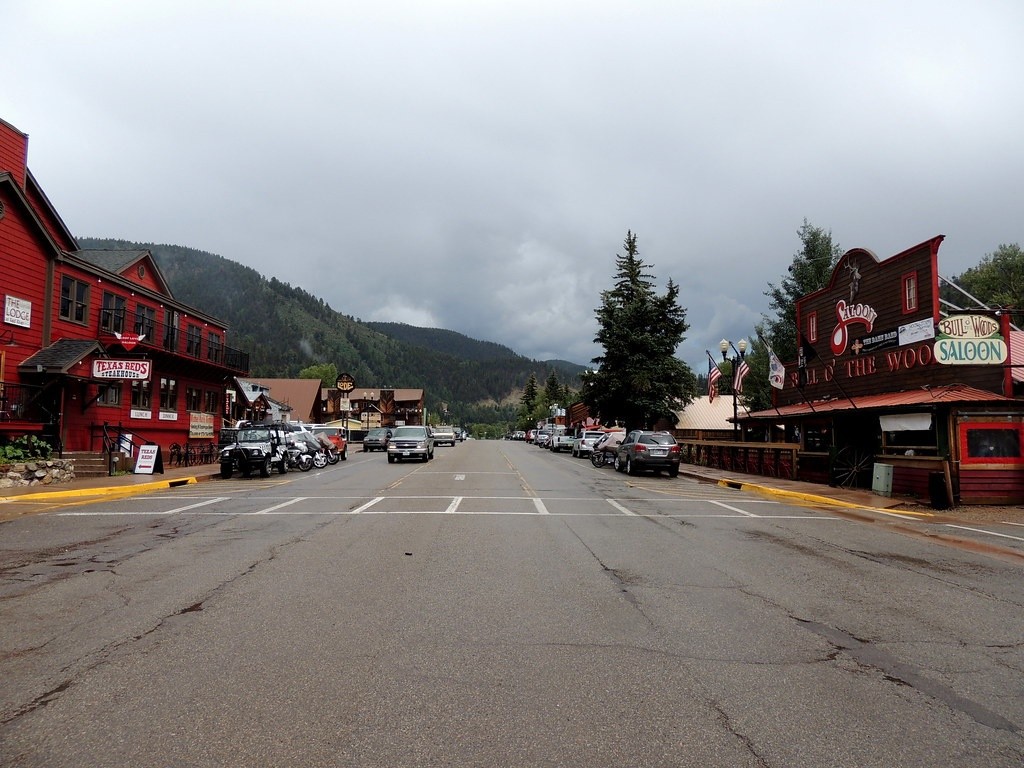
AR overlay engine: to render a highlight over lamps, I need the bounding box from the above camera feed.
[788,252,850,273]
[0,330,19,347]
[922,384,948,399]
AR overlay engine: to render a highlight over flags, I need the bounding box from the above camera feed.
[708,356,722,405]
[797,332,818,388]
[763,340,785,390]
[733,354,750,393]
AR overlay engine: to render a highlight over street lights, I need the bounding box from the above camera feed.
[363,392,374,434]
[527,417,533,429]
[549,403,558,437]
[719,339,747,442]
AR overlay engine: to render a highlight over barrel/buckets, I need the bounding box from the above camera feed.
[928,471,959,510]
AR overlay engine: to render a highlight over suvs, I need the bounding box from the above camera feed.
[219,424,289,479]
[613,430,681,478]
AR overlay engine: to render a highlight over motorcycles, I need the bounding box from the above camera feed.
[297,431,328,469]
[287,432,313,471]
[315,431,339,464]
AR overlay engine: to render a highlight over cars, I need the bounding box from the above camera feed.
[571,431,605,458]
[550,427,578,452]
[363,428,393,452]
[525,429,537,444]
[387,426,434,463]
[453,428,468,442]
[534,430,553,445]
[512,431,526,440]
[293,424,326,433]
[311,427,348,461]
[432,426,456,447]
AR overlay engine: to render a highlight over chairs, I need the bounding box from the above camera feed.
[169,441,219,465]
